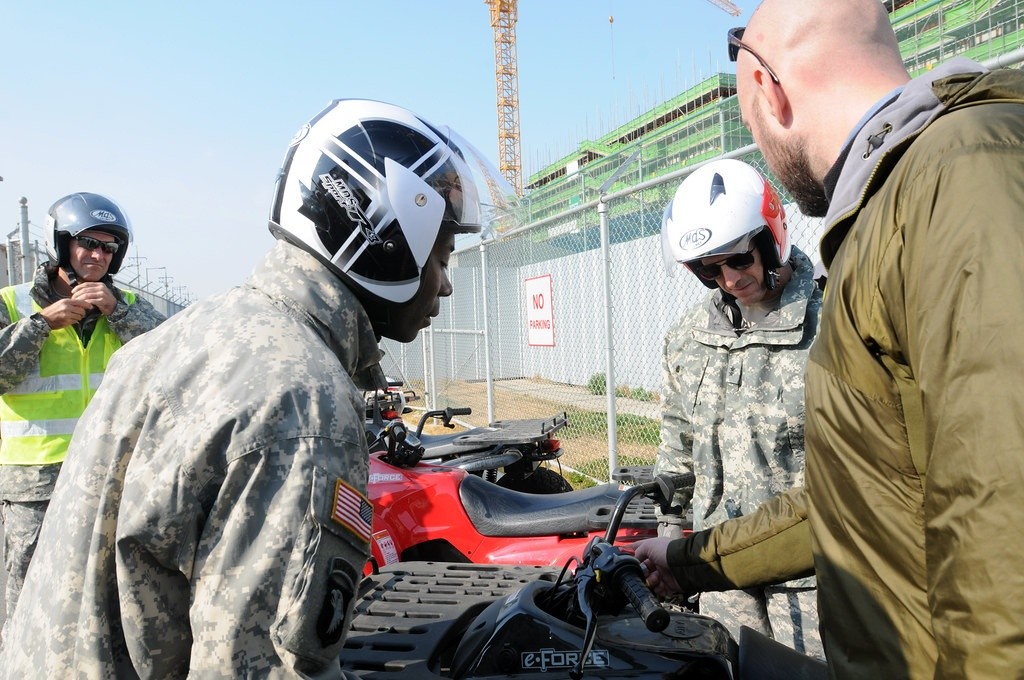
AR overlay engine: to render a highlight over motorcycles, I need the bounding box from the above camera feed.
[336,366,831,680]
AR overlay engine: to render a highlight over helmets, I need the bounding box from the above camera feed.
[48,192,129,274]
[268,98,481,311]
[667,159,791,289]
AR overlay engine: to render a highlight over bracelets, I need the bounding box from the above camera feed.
[658,522,684,527]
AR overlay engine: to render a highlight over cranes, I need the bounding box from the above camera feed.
[483,0,743,219]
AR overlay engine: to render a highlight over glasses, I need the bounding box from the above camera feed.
[726,28,778,84]
[71,234,120,254]
[695,242,756,280]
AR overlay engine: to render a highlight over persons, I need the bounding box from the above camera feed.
[653,158,827,662]
[0,98,482,680]
[0,191,169,655]
[625,0,1024,680]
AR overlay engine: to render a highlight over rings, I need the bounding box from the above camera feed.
[646,579,650,588]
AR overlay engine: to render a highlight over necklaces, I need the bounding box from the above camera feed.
[51,279,73,298]
